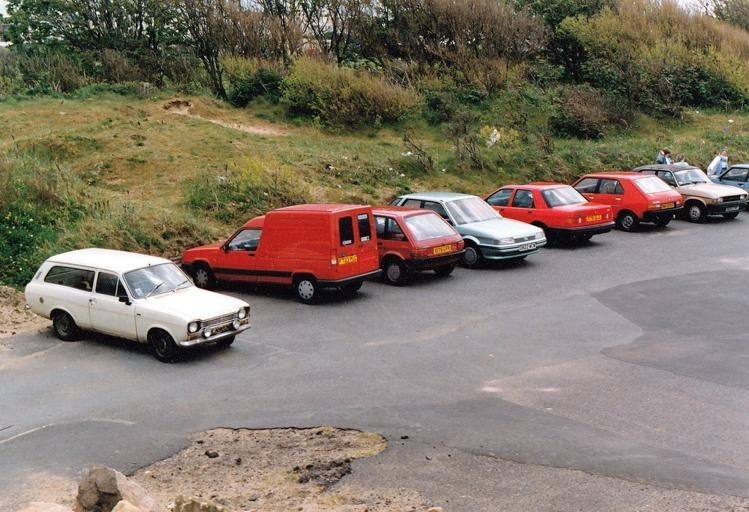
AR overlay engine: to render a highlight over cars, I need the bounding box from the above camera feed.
[480,182,616,250]
[569,170,684,234]
[632,163,748,223]
[709,164,748,195]
[22,246,251,364]
[387,192,548,270]
[368,204,464,286]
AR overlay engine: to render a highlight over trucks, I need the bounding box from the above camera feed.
[177,201,382,305]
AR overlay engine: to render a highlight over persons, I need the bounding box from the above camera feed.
[667,154,689,180]
[659,148,672,164]
[715,148,730,175]
